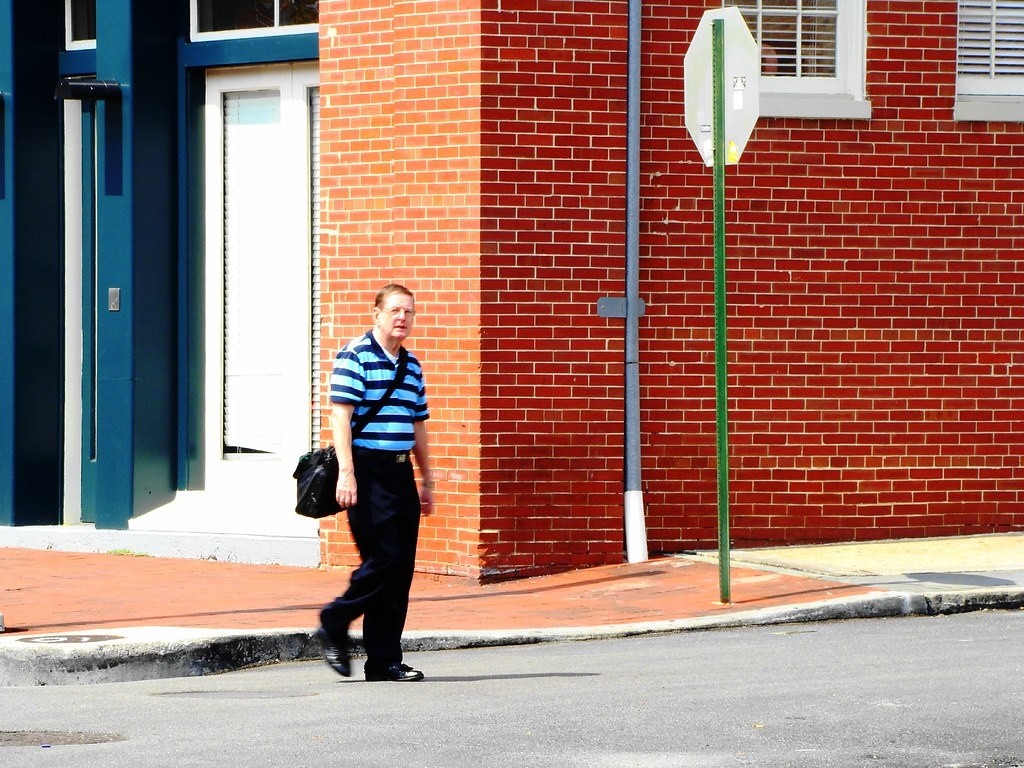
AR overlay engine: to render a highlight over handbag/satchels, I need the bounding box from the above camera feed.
[293,446,347,518]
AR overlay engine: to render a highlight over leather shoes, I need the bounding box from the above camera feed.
[317,627,351,677]
[365,664,424,681]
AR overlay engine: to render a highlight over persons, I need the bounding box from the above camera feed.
[314,284,436,682]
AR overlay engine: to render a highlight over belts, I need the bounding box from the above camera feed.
[379,451,413,463]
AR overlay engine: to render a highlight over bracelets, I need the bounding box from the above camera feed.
[422,483,434,488]
